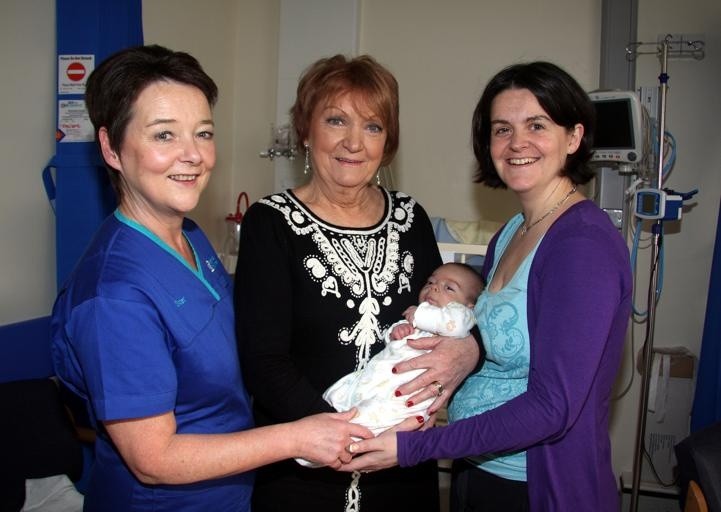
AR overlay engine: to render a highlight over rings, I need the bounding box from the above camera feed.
[433,381,442,392]
[365,469,369,473]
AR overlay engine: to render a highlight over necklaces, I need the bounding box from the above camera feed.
[520,184,577,236]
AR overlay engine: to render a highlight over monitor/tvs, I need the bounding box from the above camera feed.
[587,90,643,164]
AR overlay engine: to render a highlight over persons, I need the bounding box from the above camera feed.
[295,262,486,468]
[335,62,633,512]
[234,56,487,511]
[48,45,375,511]
